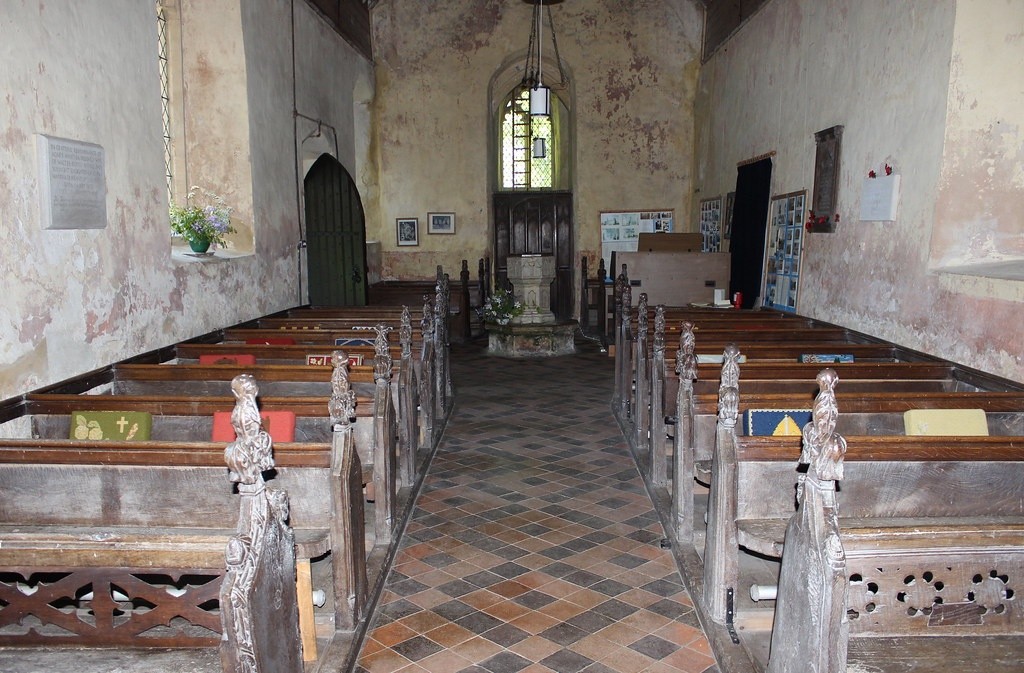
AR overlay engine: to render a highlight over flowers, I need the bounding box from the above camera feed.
[474,288,526,324]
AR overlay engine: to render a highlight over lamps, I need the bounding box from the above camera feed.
[520,0,566,118]
[532,137,546,158]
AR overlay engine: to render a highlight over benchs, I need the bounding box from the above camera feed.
[0,263,1024,673]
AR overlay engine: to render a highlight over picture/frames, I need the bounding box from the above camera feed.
[426,212,456,235]
[395,217,419,247]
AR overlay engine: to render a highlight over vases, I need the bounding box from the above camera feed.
[496,319,509,325]
